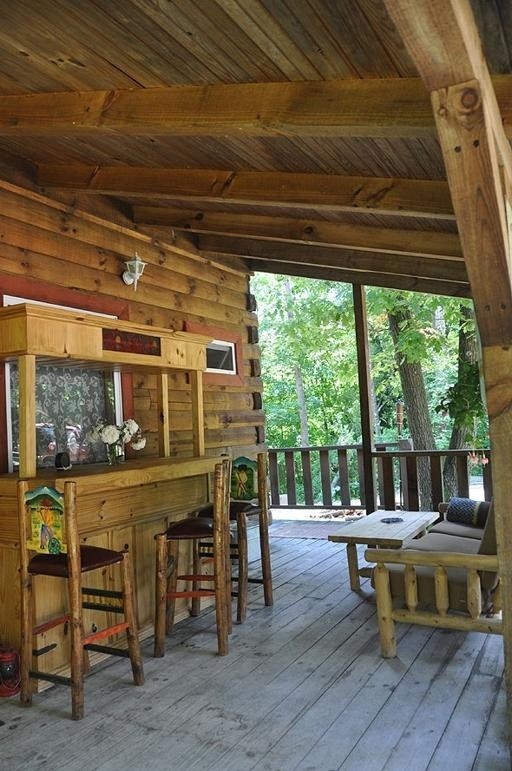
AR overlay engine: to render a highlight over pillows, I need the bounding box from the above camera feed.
[446,497,490,528]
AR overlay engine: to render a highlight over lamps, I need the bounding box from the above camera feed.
[122,253,147,287]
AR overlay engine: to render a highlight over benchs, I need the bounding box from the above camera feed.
[364,498,512,660]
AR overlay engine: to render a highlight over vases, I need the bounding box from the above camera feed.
[106,444,126,465]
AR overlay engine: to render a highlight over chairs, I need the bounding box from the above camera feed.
[154,459,233,658]
[17,481,145,721]
[191,452,273,623]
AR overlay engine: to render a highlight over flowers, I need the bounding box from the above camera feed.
[96,418,147,452]
